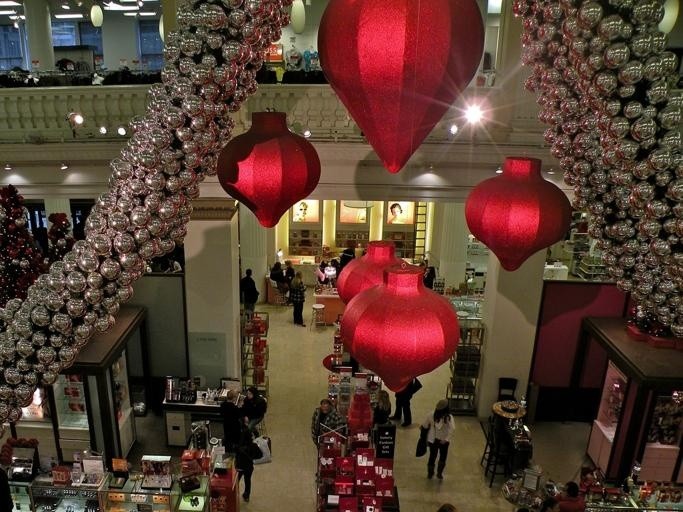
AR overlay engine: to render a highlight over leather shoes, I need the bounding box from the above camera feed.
[390,416,401,420]
[401,421,411,426]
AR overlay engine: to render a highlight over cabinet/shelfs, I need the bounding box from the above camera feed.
[584,346,683,488]
[240,310,270,394]
[574,254,608,283]
[445,317,485,417]
[43,345,138,466]
[165,412,193,445]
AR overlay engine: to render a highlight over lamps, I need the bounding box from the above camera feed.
[89,0,104,28]
[656,1,679,35]
[324,266,337,291]
[289,1,305,35]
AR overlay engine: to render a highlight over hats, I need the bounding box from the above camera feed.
[436,398,449,410]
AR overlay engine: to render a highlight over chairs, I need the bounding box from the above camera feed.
[254,395,268,438]
[268,278,288,306]
[480,377,533,489]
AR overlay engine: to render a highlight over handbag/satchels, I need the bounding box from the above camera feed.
[252,434,272,464]
[414,379,422,393]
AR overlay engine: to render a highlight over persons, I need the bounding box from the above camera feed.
[553,480,584,511]
[539,497,559,511]
[437,502,457,512]
[423,266,437,290]
[310,399,344,477]
[240,386,266,438]
[219,389,244,452]
[241,247,370,328]
[387,375,415,427]
[371,389,391,456]
[294,201,309,223]
[417,398,457,480]
[232,427,262,503]
[389,203,405,224]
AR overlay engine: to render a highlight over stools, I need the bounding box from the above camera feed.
[309,304,328,330]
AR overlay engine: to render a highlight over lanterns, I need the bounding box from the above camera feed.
[462,154,576,273]
[316,0,484,174]
[337,264,459,391]
[215,110,320,228]
[336,239,411,305]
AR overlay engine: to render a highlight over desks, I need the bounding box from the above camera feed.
[162,390,224,446]
